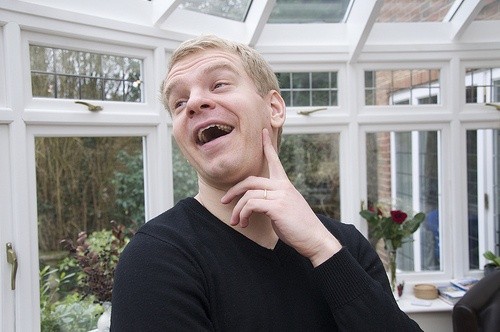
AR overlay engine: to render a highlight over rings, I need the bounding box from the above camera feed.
[264,190,267,199]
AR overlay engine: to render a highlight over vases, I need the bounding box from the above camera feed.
[385,246,401,301]
[359,200,425,249]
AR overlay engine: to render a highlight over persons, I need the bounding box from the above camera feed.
[110,36,425,332]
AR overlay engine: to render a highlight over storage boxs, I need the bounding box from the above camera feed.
[414,284,438,302]
